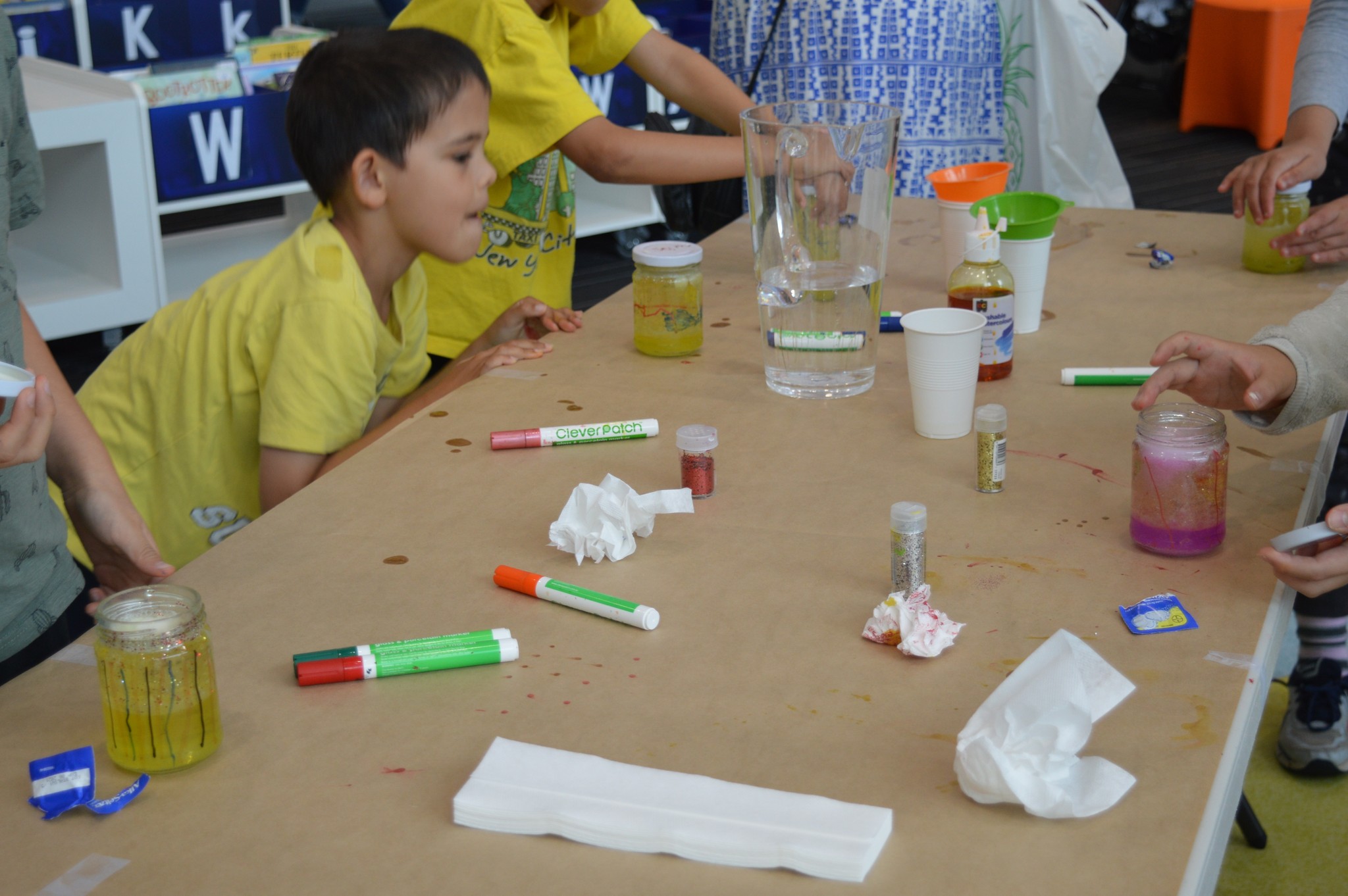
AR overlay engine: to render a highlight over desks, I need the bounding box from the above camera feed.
[0,187,1348,896]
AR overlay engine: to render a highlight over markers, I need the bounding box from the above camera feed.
[1061,367,1159,386]
[491,418,658,450]
[297,638,518,686]
[769,328,867,351]
[493,566,660,630]
[294,628,512,679]
[879,310,905,334]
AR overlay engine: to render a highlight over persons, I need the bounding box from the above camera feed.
[1134,1,1348,777]
[48,26,585,607]
[0,11,173,689]
[709,1,1006,202]
[390,1,854,387]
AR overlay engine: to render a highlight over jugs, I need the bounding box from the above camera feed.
[742,100,903,402]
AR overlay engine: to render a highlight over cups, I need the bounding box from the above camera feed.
[941,200,972,286]
[899,307,987,439]
[999,233,1056,335]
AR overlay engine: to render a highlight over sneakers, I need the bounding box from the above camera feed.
[1277,663,1348,775]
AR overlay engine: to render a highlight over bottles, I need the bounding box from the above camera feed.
[94,585,219,775]
[945,206,1014,382]
[1129,402,1230,557]
[974,403,1008,494]
[789,184,840,307]
[675,425,719,498]
[632,240,704,358]
[890,500,928,597]
[1241,180,1312,274]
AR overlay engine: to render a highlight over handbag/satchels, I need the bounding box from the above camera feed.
[645,112,741,231]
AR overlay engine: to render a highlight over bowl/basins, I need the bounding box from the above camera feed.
[969,190,1075,240]
[923,162,1014,201]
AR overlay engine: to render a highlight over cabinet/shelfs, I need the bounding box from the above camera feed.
[0,48,322,362]
